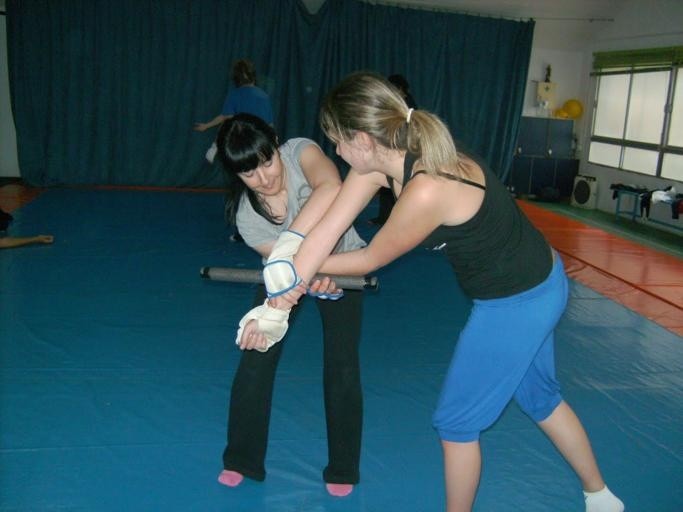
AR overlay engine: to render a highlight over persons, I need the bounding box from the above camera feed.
[217,113,368,496]
[367,75,418,225]
[0,234,53,248]
[193,60,274,242]
[234,75,625,512]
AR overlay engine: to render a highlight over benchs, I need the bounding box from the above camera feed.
[613,187,683,231]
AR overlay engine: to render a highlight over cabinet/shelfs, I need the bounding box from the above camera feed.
[509,116,580,197]
